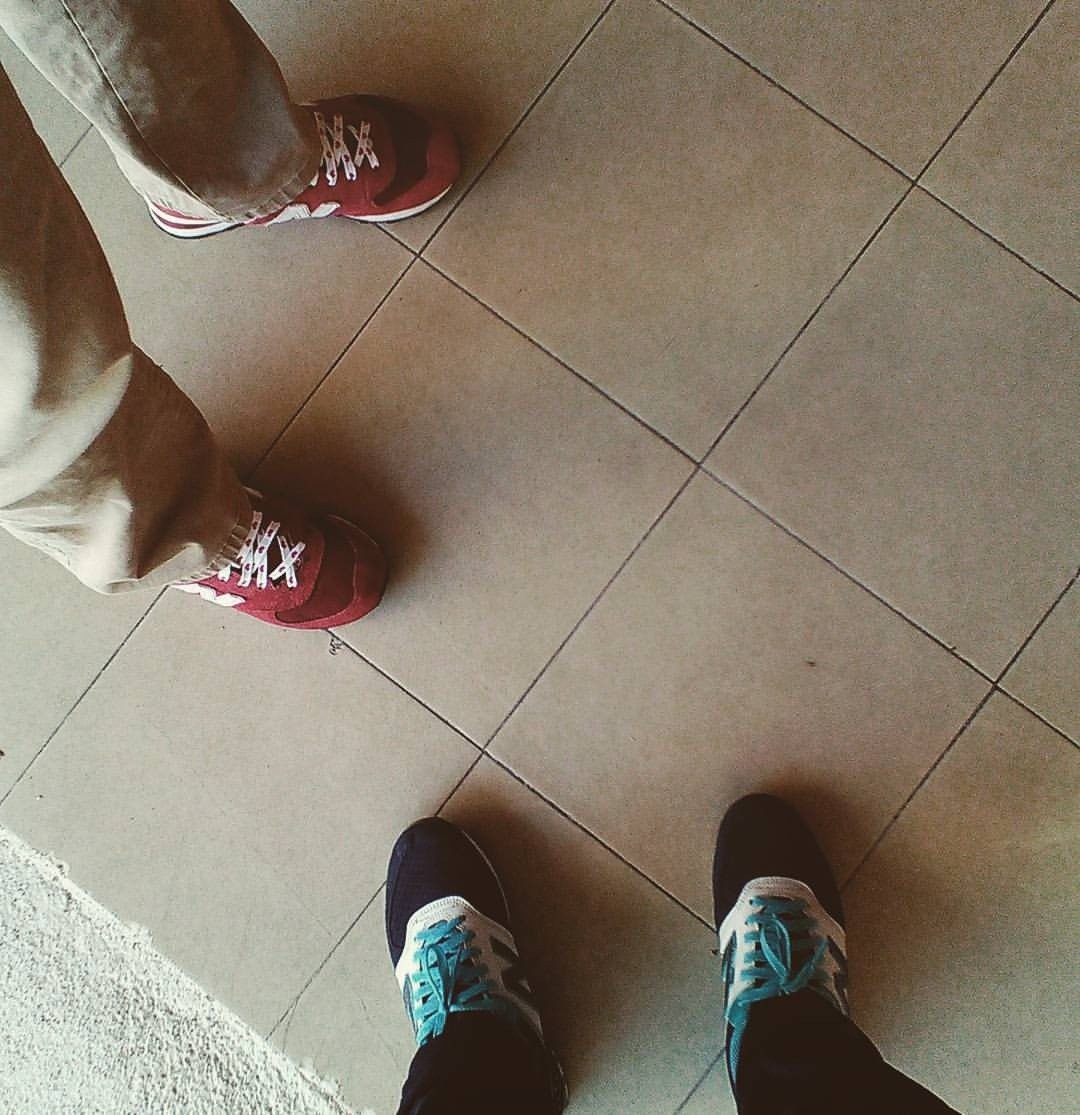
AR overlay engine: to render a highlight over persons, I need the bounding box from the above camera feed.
[384,794,963,1115]
[1,0,461,631]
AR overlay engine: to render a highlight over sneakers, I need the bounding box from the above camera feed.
[168,487,390,629]
[711,791,848,1107]
[146,94,460,240]
[386,816,569,1106]
